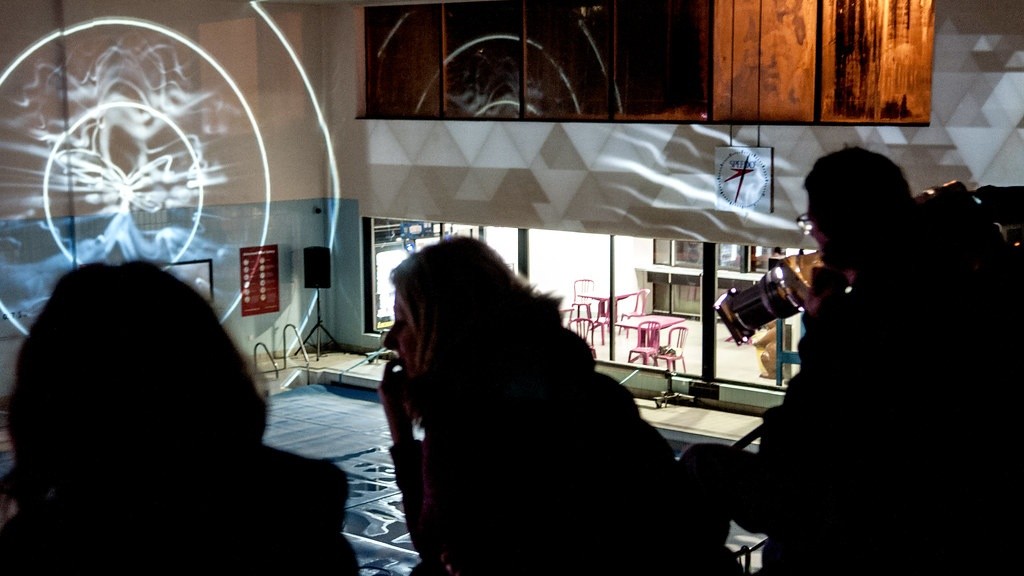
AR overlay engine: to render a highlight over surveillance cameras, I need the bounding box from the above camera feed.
[313,207,322,214]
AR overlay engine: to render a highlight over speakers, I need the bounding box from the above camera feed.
[303,246,331,288]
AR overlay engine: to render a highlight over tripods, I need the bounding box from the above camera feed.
[294,287,346,364]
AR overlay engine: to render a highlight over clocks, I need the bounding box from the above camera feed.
[714,146,773,214]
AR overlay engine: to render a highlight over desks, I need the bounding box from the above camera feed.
[577,289,638,334]
[614,316,685,366]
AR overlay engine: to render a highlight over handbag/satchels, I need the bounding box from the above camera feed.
[650,346,676,359]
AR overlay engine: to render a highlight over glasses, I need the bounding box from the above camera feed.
[797,213,816,230]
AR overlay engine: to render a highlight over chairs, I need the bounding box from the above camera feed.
[628,321,661,366]
[653,327,689,371]
[572,318,597,358]
[569,279,595,319]
[618,289,650,338]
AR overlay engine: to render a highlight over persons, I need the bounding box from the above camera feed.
[444,230,452,240]
[0,259,363,576]
[378,233,747,576]
[771,247,785,259]
[675,146,1024,576]
